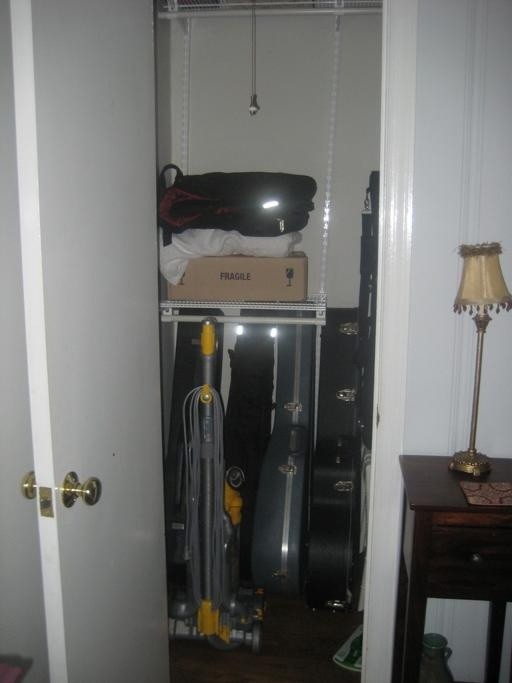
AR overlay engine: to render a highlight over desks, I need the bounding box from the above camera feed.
[389,456,510,682]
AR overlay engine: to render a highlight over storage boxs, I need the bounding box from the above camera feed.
[166,253,309,304]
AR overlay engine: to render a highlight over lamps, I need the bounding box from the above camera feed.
[450,240,512,481]
